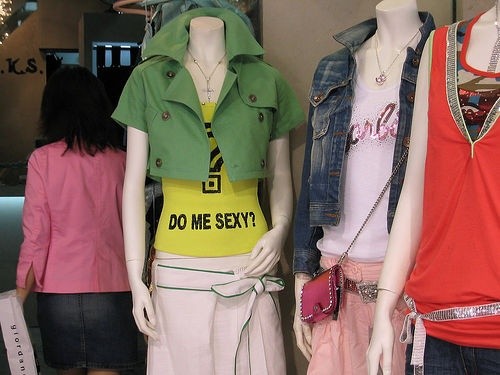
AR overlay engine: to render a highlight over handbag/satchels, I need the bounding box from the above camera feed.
[300,264,347,323]
[0,288,38,375]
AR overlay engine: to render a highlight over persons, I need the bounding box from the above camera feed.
[112,6,305,375]
[365,0,500,375]
[292,1,437,375]
[14,65,151,374]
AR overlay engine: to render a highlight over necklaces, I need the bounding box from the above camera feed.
[184,45,226,102]
[488,0,500,71]
[374,24,424,85]
